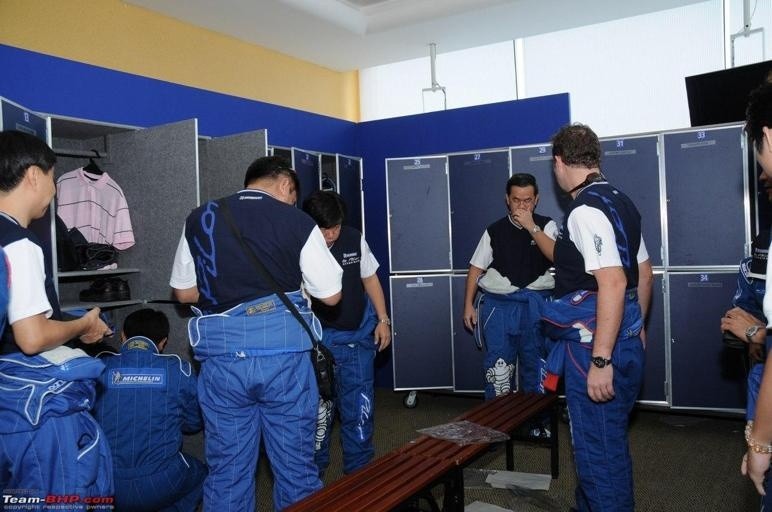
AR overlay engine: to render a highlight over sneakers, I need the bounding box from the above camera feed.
[532,424,553,443]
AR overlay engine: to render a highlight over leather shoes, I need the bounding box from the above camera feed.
[79,277,116,302]
[112,277,132,301]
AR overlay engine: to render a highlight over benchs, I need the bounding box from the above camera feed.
[279,390,558,511]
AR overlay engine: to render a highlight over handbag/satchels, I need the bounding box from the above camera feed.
[311,341,338,403]
[62,242,121,272]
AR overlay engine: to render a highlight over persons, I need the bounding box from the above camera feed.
[720,169,772,421]
[301,190,392,478]
[550,121,654,511]
[463,173,559,446]
[0,129,117,511]
[740,76,772,512]
[170,156,344,511]
[91,307,207,509]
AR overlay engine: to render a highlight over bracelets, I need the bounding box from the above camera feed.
[744,418,772,454]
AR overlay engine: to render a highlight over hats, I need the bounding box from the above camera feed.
[745,230,772,281]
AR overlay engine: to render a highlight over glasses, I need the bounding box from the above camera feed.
[570,172,608,193]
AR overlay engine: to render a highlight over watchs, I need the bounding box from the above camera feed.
[744,319,764,342]
[532,223,541,235]
[591,351,611,369]
[379,315,392,328]
